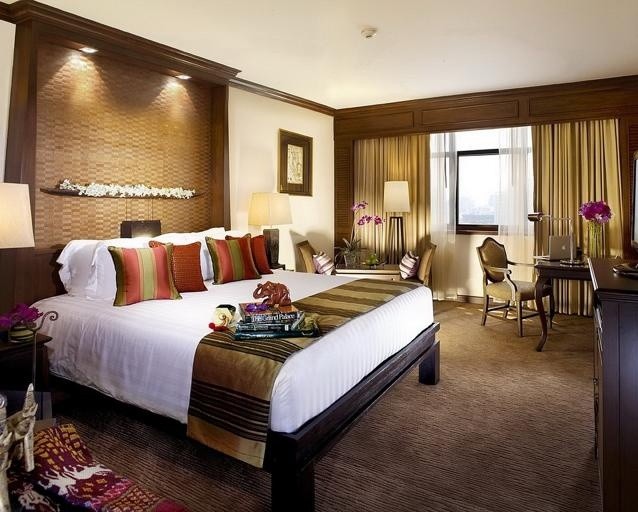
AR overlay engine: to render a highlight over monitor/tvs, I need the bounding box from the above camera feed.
[630,150,638,250]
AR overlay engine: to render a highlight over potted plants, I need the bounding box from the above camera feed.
[334,226,361,269]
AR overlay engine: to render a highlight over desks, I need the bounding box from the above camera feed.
[533,258,593,352]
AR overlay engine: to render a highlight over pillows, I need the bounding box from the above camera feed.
[107,243,182,305]
[312,250,336,276]
[85,241,151,301]
[398,250,420,280]
[205,233,262,285]
[152,225,225,282]
[225,235,273,275]
[149,240,208,292]
[56,235,151,298]
[226,229,263,238]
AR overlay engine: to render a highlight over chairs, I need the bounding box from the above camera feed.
[296,240,318,274]
[391,242,437,297]
[476,237,555,337]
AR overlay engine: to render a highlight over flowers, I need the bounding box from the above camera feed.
[0,302,44,330]
[351,200,383,226]
[577,199,613,253]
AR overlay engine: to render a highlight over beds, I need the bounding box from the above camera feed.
[0,242,440,512]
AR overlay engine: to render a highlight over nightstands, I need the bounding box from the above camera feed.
[1,333,52,421]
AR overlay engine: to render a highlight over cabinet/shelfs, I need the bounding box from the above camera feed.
[586,256,637,512]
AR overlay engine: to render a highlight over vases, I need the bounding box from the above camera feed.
[588,221,605,259]
[8,323,35,343]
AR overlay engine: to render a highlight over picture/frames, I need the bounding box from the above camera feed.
[276,127,313,197]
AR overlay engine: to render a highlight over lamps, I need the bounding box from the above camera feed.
[383,181,410,264]
[248,192,292,269]
[0,183,36,249]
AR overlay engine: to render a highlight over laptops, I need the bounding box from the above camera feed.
[533,236,576,261]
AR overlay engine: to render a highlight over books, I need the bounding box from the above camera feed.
[233,301,318,340]
